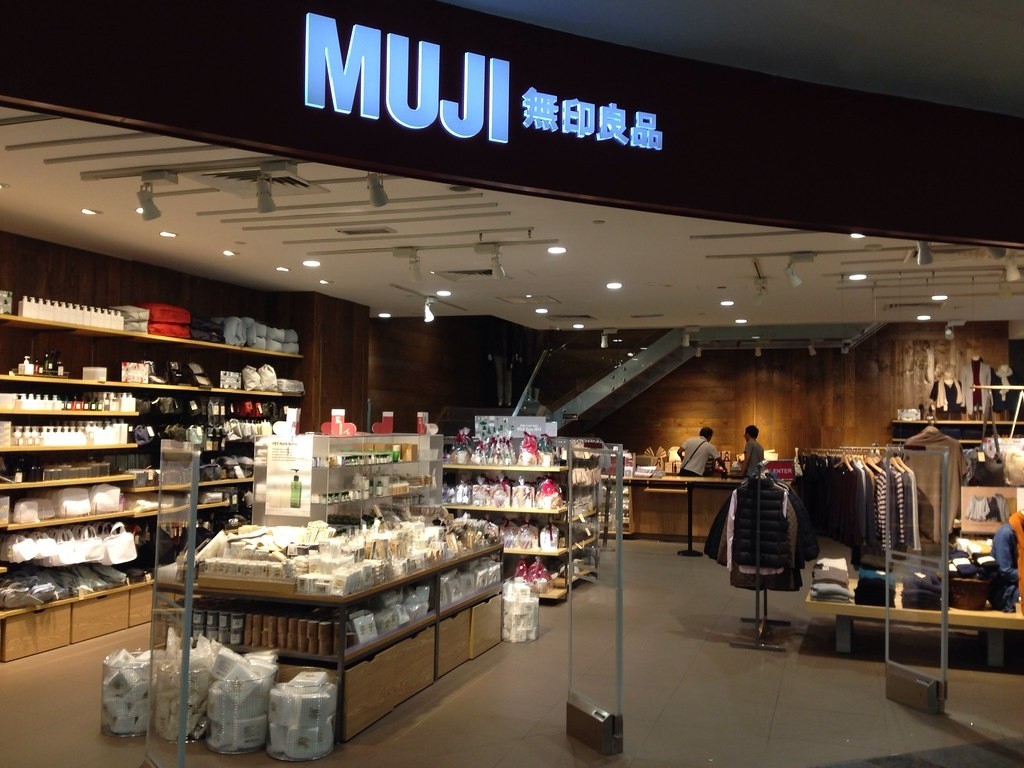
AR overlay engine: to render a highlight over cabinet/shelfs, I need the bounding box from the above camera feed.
[549,508,597,586]
[891,420,1024,443]
[0,313,305,662]
[152,542,504,745]
[442,438,570,603]
[252,433,445,529]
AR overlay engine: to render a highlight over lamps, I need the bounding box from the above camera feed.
[490,252,505,281]
[755,346,761,356]
[681,332,690,346]
[916,241,933,265]
[367,172,389,207]
[695,346,701,357]
[423,302,434,323]
[783,262,802,288]
[408,256,422,284]
[255,177,277,213]
[808,343,816,356]
[600,333,608,348]
[136,183,161,221]
[945,326,955,341]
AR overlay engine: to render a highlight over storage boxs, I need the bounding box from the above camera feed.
[665,461,681,473]
[363,443,375,450]
[636,455,665,471]
[375,443,392,450]
[402,445,417,461]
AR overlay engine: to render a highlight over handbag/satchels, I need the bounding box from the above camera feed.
[0,522,138,568]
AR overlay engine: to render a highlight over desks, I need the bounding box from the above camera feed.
[805,577,1024,654]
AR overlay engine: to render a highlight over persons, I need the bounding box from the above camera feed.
[987,508,1024,613]
[992,365,1017,413]
[737,425,764,478]
[676,427,728,477]
[929,373,961,412]
[961,355,993,415]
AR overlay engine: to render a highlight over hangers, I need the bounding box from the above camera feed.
[905,416,949,445]
[741,460,789,494]
[792,446,911,476]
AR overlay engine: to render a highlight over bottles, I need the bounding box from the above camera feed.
[597,512,629,532]
